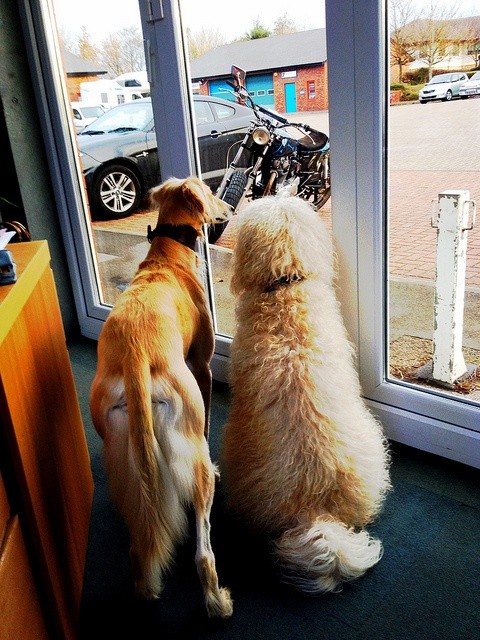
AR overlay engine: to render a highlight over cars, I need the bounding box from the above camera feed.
[459,71,480,99]
[419,73,469,104]
[76,96,295,218]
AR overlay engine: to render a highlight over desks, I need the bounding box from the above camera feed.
[1,240,95,640]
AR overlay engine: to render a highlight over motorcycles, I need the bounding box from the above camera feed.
[202,64,331,244]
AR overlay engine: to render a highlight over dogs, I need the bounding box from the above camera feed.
[221,177,394,593]
[90,177,235,618]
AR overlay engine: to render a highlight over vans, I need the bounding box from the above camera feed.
[71,101,105,134]
